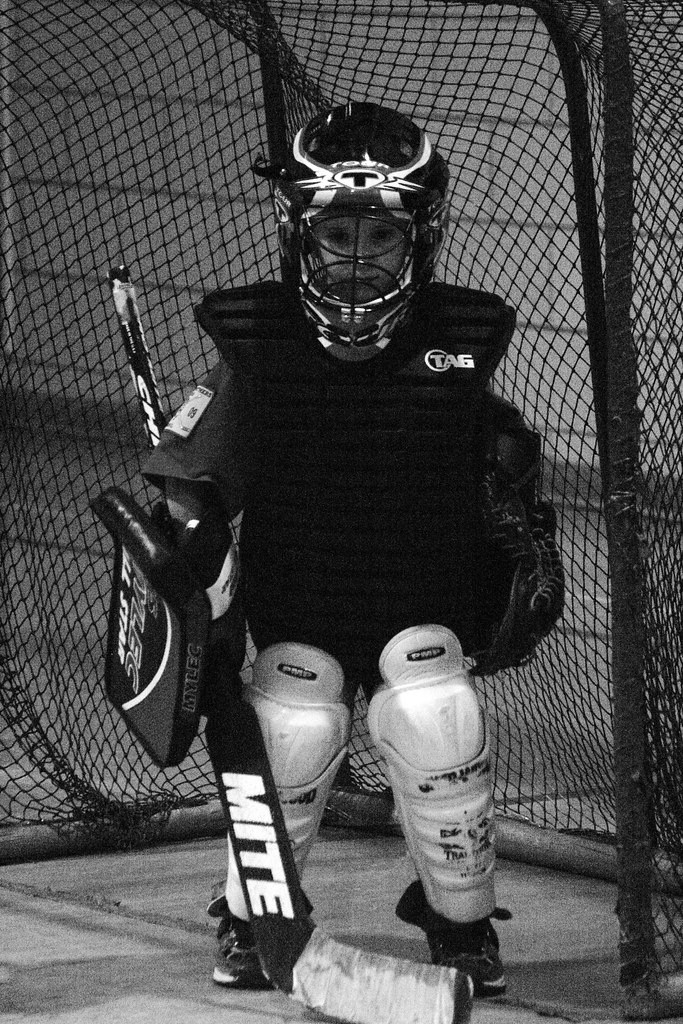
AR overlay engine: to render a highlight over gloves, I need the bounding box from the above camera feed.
[150,502,246,674]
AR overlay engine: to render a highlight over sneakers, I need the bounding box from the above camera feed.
[396,881,511,995]
[207,896,313,989]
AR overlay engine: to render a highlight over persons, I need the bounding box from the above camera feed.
[91,104,566,995]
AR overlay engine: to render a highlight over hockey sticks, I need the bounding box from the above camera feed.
[108,263,475,1024]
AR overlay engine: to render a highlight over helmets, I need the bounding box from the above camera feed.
[273,104,449,360]
